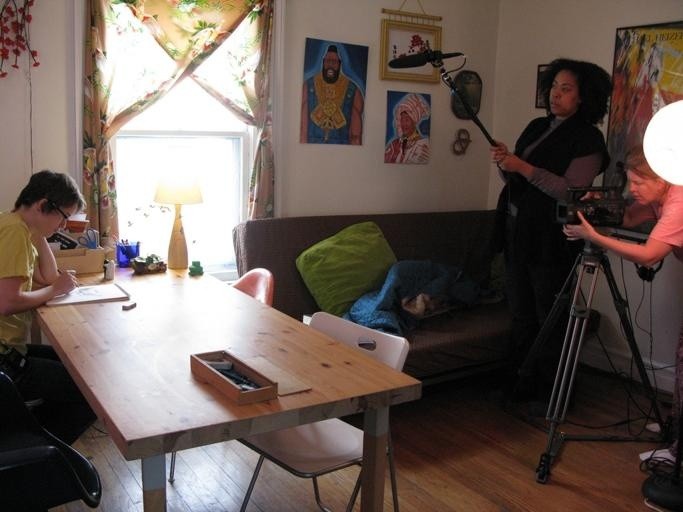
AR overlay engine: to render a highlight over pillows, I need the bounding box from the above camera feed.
[295,221,399,317]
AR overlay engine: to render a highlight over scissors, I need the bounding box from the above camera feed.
[77,229,96,249]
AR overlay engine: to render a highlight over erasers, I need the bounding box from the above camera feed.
[122,302,137,310]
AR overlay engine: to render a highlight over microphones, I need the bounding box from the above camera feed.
[389,50,463,68]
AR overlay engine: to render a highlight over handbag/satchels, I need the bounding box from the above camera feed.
[493,181,524,233]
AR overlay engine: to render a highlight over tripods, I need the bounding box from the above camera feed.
[509,240,673,484]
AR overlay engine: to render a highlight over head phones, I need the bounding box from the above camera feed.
[634,239,664,281]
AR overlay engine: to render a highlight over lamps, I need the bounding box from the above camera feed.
[153,183,203,270]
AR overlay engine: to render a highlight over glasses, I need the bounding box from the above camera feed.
[56,206,70,222]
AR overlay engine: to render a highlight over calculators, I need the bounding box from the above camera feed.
[47,233,77,250]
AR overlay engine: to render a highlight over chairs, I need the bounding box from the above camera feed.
[0,371,102,512]
[230,268,275,307]
[168,311,411,512]
[21,392,43,407]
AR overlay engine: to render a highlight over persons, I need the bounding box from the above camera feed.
[299,45,363,144]
[563,146,682,463]
[488,58,614,405]
[0,169,97,443]
[384,94,428,162]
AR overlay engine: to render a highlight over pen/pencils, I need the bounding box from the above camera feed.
[56,270,80,289]
[219,369,262,388]
[111,234,140,262]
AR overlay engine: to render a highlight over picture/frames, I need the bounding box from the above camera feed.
[535,64,550,108]
[378,18,443,84]
[604,21,683,233]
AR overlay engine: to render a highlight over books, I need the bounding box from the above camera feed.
[45,283,130,307]
[57,212,87,233]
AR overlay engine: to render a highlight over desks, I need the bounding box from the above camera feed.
[31,262,422,512]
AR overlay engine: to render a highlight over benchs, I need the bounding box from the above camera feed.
[231,209,521,386]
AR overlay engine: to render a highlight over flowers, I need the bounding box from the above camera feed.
[0,0,40,77]
[408,35,430,53]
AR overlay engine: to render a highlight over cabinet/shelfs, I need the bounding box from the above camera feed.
[571,227,682,394]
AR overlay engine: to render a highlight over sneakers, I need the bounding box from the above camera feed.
[640,448,676,464]
[645,421,668,433]
